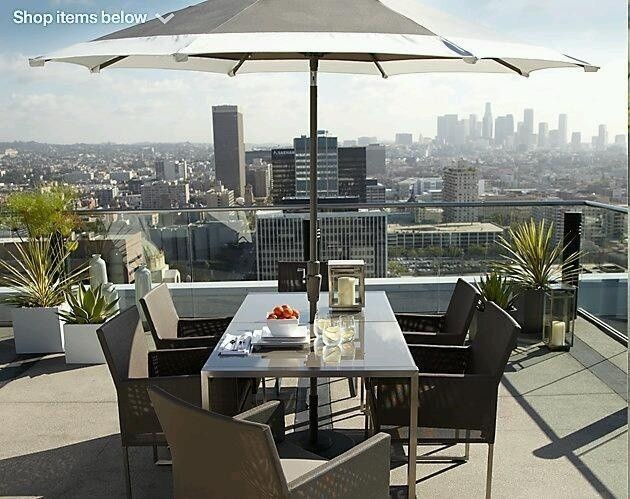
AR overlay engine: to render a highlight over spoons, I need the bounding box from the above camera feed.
[221,334,252,351]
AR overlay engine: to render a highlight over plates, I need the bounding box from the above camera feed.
[248,326,311,348]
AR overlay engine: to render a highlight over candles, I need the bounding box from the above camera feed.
[337,275,355,308]
[550,318,565,345]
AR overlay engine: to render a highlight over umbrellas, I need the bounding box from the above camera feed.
[28,0,601,445]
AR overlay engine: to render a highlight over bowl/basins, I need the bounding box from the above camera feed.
[265,310,300,336]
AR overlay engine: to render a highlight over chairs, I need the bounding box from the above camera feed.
[138,281,264,412]
[363,291,523,498]
[97,303,263,498]
[272,256,360,400]
[139,375,394,499]
[361,274,485,448]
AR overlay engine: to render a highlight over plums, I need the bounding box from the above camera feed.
[269,305,299,320]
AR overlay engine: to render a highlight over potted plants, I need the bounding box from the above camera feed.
[0,176,94,358]
[468,267,523,350]
[54,269,122,367]
[490,217,589,339]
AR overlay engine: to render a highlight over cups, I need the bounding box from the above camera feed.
[313,337,355,367]
[312,314,356,344]
